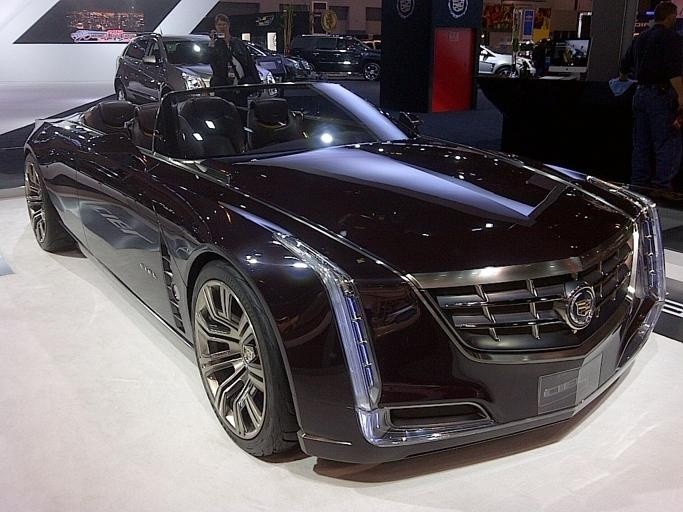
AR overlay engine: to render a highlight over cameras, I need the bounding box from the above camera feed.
[214,33,226,39]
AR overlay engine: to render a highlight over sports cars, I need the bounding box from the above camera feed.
[20,80,666,464]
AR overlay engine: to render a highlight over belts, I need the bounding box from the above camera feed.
[637,83,674,90]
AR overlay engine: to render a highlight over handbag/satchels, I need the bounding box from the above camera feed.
[236,74,261,98]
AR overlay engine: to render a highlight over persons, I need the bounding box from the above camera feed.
[208,14,259,94]
[618,2,683,192]
[534,38,549,75]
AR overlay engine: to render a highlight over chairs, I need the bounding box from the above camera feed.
[180,92,248,157]
[132,101,161,151]
[245,96,307,152]
[84,99,134,142]
